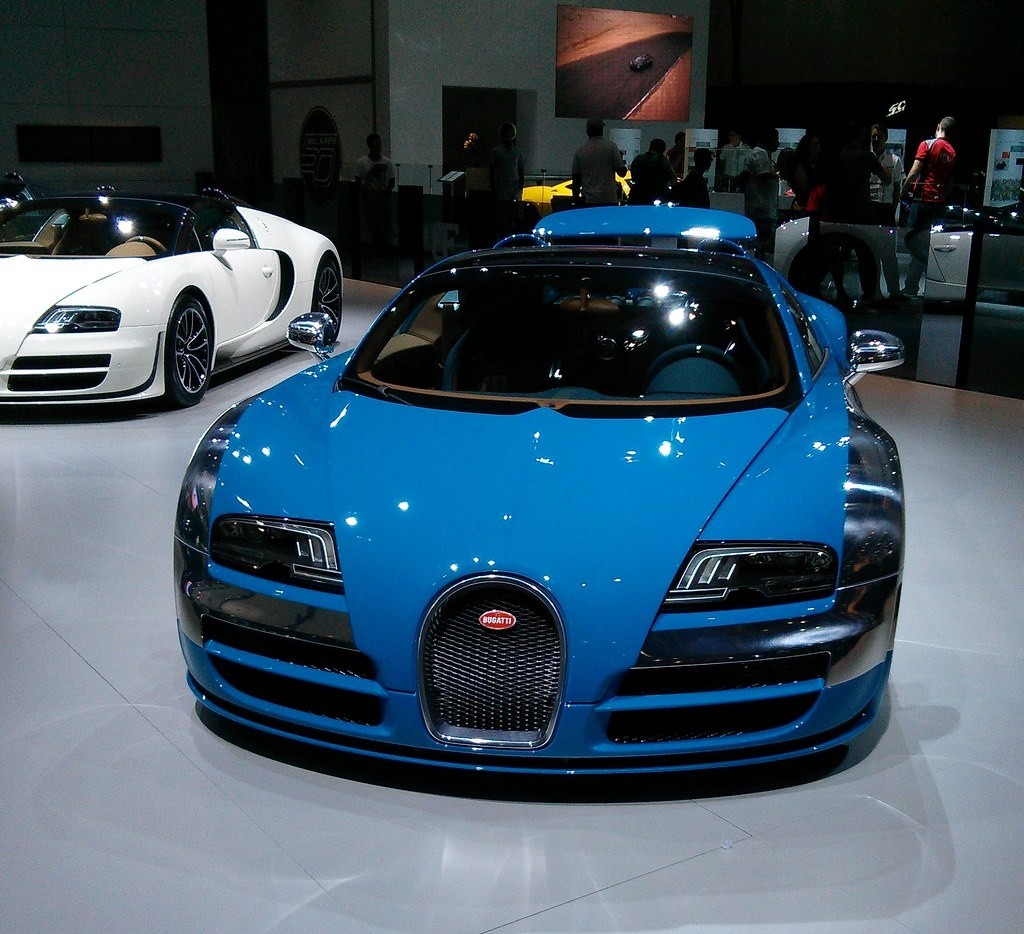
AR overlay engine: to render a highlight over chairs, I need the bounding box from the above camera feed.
[619,264,768,387]
[442,273,573,398]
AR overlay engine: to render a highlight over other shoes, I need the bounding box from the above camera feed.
[889,292,912,302]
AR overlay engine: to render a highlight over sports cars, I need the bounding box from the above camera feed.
[776,186,1023,307]
[174,203,905,800]
[0,171,343,408]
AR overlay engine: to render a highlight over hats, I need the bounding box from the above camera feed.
[586,117,606,129]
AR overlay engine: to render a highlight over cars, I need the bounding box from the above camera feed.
[522,169,631,217]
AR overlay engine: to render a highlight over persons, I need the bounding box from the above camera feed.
[630,115,959,304]
[489,121,526,201]
[354,133,397,192]
[572,116,627,206]
